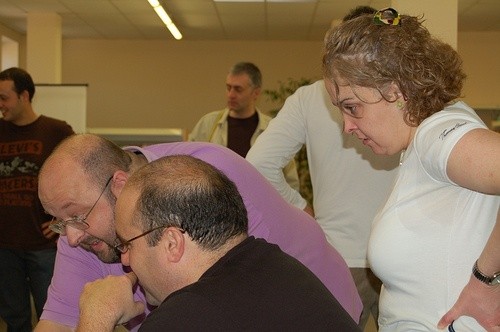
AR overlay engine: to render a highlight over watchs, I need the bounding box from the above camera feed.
[473,259,500,286]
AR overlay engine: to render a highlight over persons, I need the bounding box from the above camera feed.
[324,8,500,332]
[0,67,75,332]
[35,133,378,332]
[76,155,363,332]
[245,6,404,332]
[188,62,300,193]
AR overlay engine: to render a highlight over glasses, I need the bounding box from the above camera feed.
[49,175,112,233]
[114,225,184,254]
